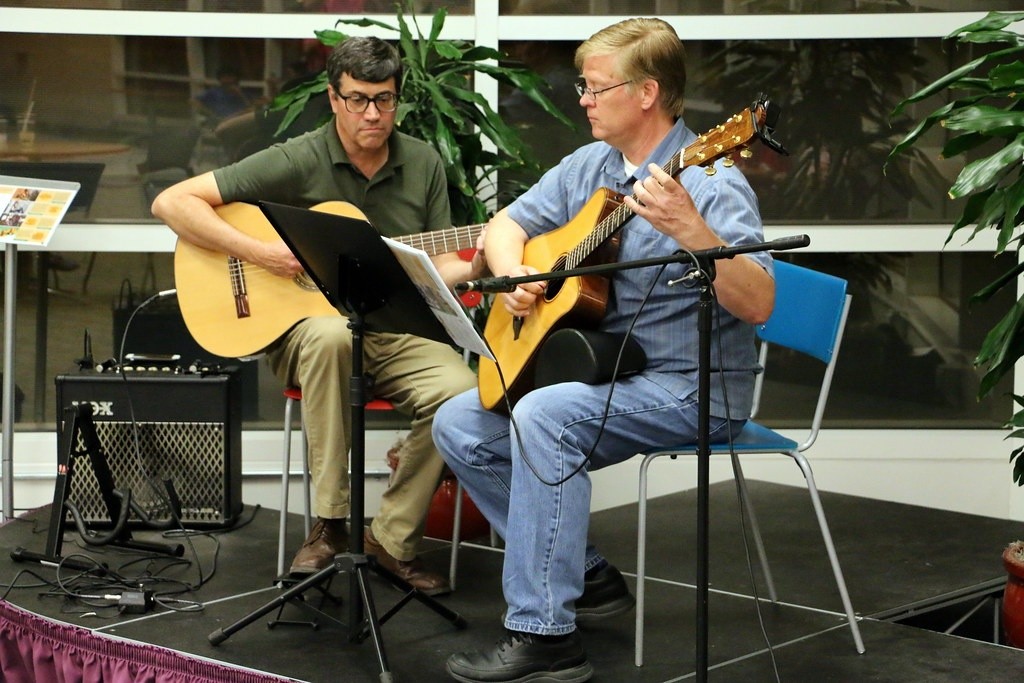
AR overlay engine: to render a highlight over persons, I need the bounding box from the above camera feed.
[152,37,488,597]
[431,19,776,683]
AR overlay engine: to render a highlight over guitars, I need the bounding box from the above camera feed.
[476,88,790,417]
[173,198,490,362]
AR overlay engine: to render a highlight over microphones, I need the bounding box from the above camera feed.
[456,276,516,293]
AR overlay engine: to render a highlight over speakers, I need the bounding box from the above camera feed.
[56,365,247,532]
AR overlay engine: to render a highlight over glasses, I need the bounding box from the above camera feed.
[332,86,401,113]
[574,76,639,99]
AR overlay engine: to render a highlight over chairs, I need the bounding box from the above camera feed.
[274,290,482,592]
[490,259,867,667]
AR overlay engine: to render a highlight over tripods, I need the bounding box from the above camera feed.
[209,200,464,683]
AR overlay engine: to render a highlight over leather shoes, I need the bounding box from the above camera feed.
[499,564,634,622]
[289,518,347,574]
[445,629,594,683]
[362,530,450,595]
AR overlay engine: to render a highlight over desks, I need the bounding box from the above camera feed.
[1,134,132,305]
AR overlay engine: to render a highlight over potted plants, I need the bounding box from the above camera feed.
[881,11,1024,650]
[262,0,577,546]
[689,0,951,384]
